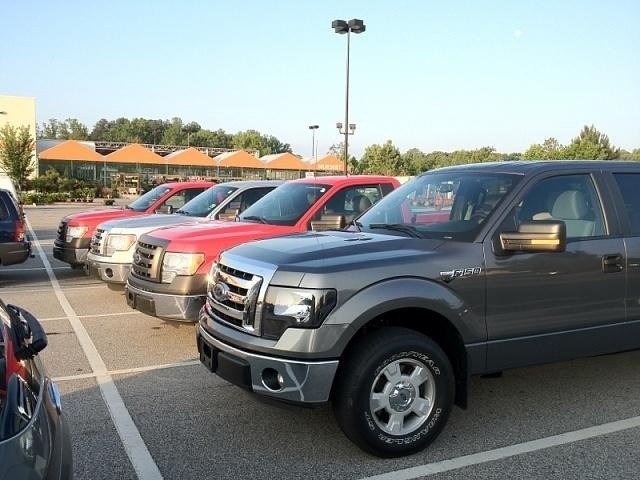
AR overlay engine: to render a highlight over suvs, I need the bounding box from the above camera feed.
[0,298,74,480]
[0,174,35,266]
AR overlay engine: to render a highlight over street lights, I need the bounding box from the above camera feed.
[308,124,320,156]
[331,18,366,176]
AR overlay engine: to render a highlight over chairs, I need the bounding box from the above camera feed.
[476,189,600,237]
[306,193,373,225]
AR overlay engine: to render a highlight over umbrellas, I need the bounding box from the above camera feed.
[103,142,169,195]
[263,152,309,180]
[217,150,266,180]
[161,146,217,181]
[309,156,350,176]
[38,138,108,178]
